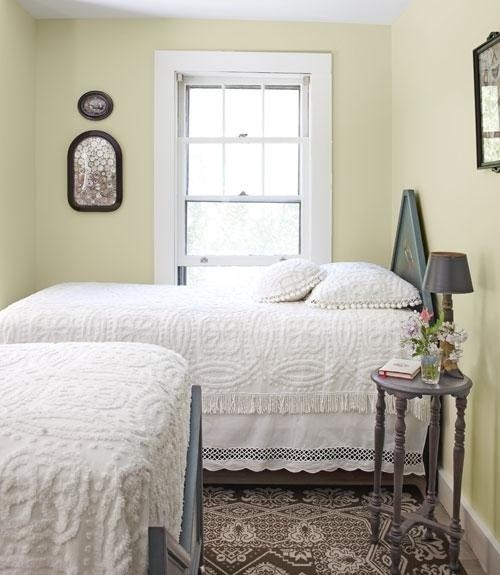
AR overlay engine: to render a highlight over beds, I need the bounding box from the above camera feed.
[0,341,206,575]
[0,189,436,500]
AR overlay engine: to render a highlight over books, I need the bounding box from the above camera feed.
[378,358,421,380]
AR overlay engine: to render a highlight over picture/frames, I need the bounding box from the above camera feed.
[67,130,122,212]
[78,91,113,121]
[473,31,500,173]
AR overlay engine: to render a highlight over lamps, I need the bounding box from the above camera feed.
[421,252,473,379]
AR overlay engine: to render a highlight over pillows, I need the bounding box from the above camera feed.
[259,257,329,303]
[302,261,423,310]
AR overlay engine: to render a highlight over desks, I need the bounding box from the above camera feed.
[368,365,472,575]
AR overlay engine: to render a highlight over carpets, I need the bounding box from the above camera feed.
[204,484,467,575]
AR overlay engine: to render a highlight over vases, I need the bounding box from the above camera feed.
[421,353,442,385]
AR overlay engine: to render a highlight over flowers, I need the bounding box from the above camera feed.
[398,306,468,380]
